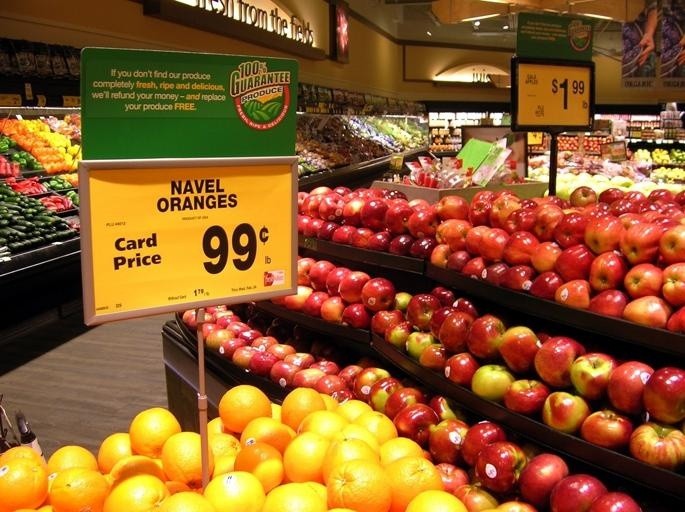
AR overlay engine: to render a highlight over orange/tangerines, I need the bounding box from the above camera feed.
[0,384,467,512]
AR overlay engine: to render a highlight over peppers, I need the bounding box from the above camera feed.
[0,133,80,230]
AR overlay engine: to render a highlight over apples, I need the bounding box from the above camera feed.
[181,186,685,512]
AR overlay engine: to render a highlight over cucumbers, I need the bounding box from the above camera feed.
[0,181,75,253]
